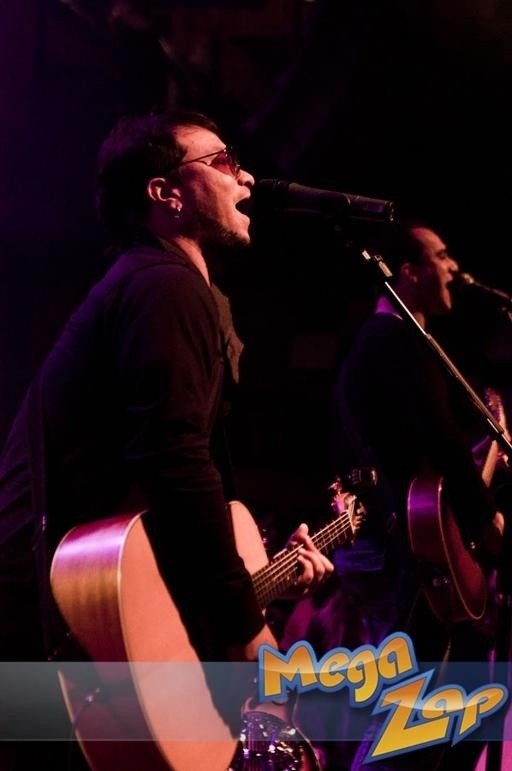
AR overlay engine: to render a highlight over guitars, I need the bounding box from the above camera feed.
[408,389,509,622]
[50,495,365,771]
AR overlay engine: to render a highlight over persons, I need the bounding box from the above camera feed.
[332,222,510,657]
[1,113,334,771]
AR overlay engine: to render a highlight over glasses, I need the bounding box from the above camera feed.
[155,148,241,180]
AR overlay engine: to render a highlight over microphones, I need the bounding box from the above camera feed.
[253,175,395,217]
[456,267,510,305]
